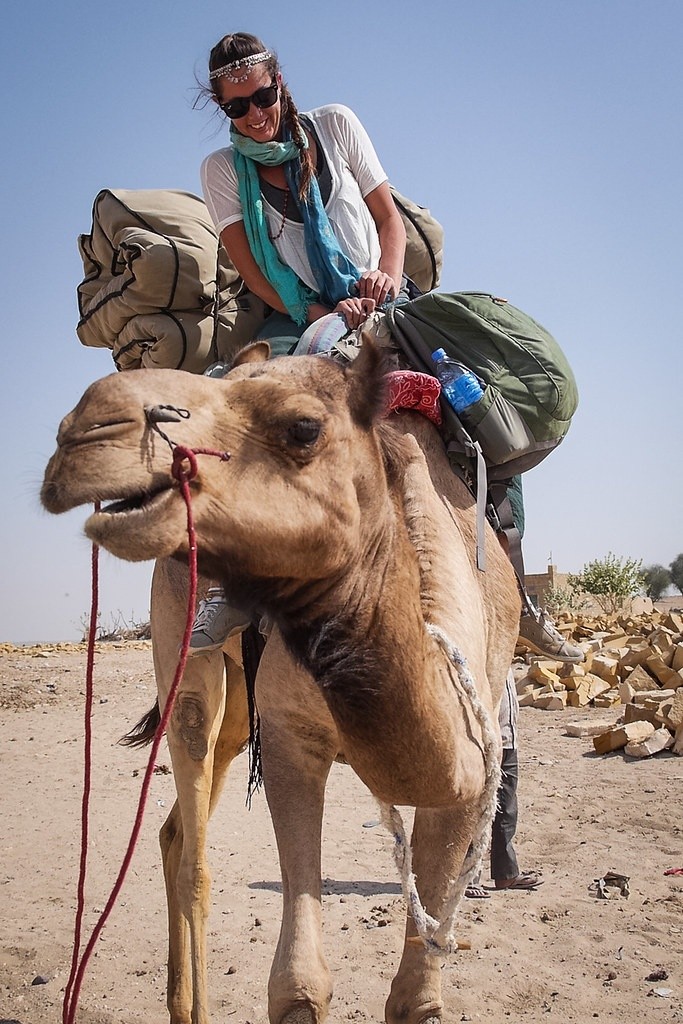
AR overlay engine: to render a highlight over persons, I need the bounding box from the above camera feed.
[460,663,545,898]
[179,29,588,665]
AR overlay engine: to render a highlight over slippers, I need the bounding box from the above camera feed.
[465,888,491,898]
[496,875,544,889]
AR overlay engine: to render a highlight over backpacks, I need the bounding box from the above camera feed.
[387,291,578,571]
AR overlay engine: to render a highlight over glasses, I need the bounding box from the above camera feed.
[214,73,278,119]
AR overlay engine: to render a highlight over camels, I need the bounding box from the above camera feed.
[26,349,522,1022]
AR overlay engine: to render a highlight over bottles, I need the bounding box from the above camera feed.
[431,348,484,414]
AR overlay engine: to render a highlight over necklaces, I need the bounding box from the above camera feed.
[259,182,289,242]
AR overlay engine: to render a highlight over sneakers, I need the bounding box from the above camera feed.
[177,589,253,657]
[518,616,585,663]
